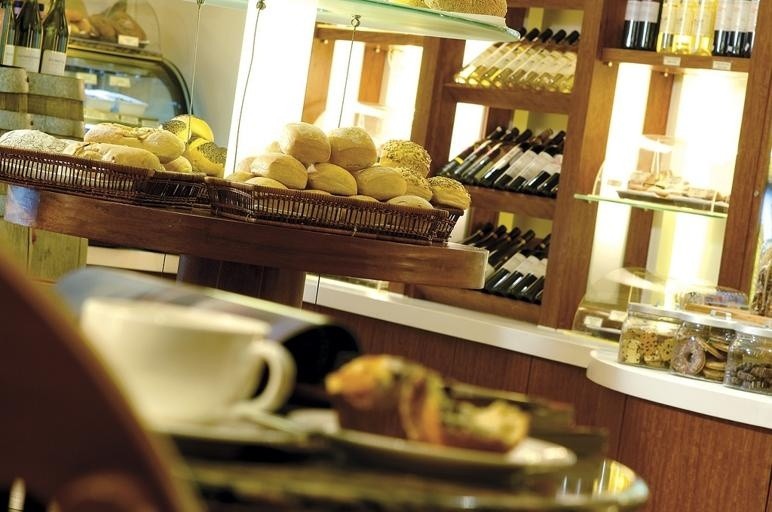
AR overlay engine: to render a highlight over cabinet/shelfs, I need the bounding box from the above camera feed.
[56,0,195,132]
[387,0,772,344]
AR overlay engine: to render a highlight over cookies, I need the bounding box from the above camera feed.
[616,236,772,395]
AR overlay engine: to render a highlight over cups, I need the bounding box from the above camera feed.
[76,291,294,421]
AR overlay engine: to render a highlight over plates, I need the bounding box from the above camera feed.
[141,424,322,461]
[287,403,577,482]
[315,2,522,43]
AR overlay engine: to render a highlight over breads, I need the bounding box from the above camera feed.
[225,122,470,227]
[1,113,227,180]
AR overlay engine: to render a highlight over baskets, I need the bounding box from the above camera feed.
[193,174,218,209]
[0,146,207,211]
[429,202,464,243]
[205,178,450,246]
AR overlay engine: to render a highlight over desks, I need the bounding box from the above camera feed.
[2,185,489,309]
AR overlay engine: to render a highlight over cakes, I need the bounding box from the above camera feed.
[321,347,533,454]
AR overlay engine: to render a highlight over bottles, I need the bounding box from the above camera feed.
[449,23,581,93]
[613,302,772,396]
[0,0,72,73]
[614,0,762,54]
[436,119,566,198]
[458,220,550,305]
